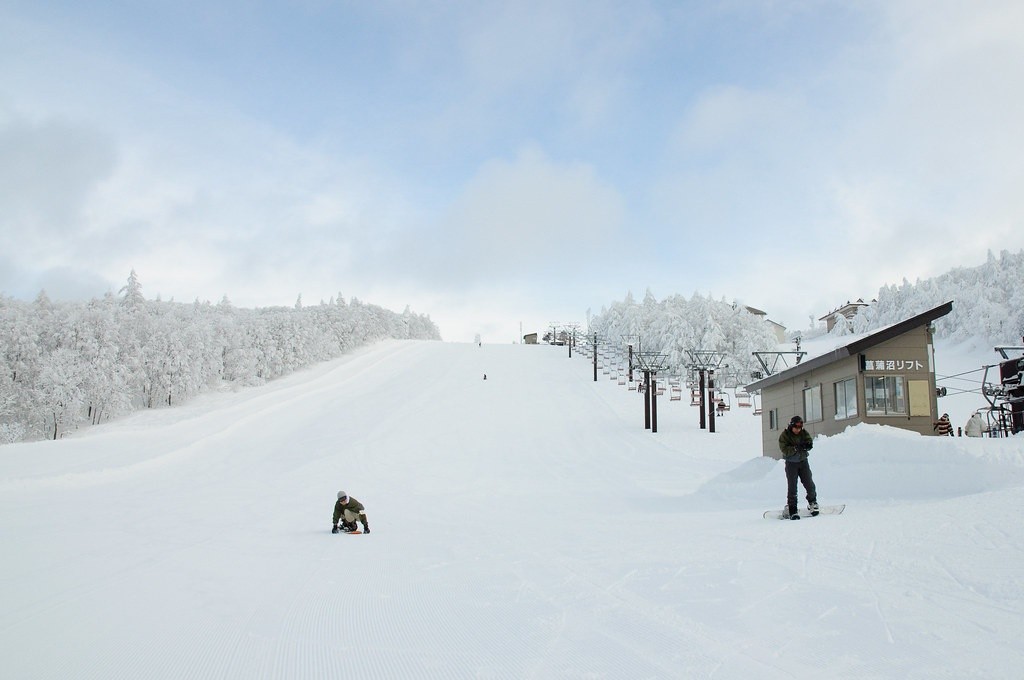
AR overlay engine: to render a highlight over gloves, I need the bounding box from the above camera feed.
[364,528,370,533]
[332,525,338,533]
[799,441,812,452]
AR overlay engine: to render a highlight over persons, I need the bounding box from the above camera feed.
[964,414,987,438]
[779,416,819,520]
[934,413,954,436]
[638,383,642,392]
[332,491,370,534]
[717,399,726,416]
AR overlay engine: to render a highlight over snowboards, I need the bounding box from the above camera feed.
[337,526,362,534]
[764,504,846,520]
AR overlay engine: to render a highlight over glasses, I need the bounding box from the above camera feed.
[338,496,347,501]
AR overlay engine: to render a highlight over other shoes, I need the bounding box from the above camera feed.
[341,524,357,532]
[338,523,350,531]
[782,505,800,520]
[807,502,819,516]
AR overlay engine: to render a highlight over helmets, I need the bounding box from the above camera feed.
[337,491,349,506]
[788,416,803,428]
